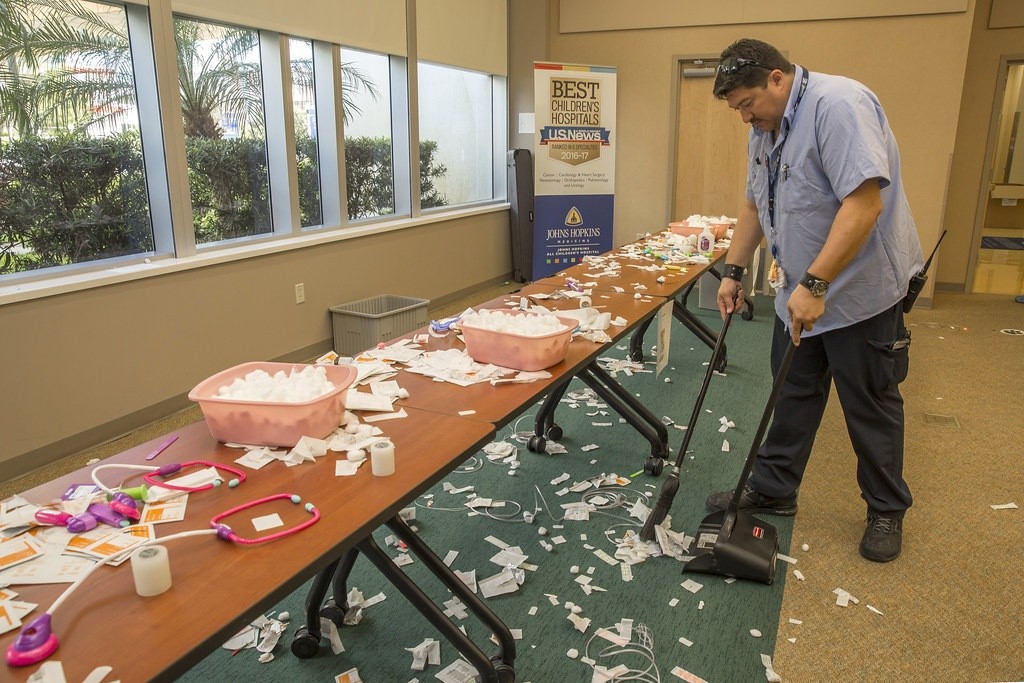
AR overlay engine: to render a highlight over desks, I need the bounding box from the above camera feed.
[2,214,756,683]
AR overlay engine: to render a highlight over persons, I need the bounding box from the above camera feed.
[702,38,926,564]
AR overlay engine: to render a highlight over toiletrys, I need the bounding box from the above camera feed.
[696,219,716,256]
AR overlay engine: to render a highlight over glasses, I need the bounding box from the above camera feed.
[714,57,775,81]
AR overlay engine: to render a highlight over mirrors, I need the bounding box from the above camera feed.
[990,52,1024,188]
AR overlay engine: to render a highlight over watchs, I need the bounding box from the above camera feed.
[799,272,831,297]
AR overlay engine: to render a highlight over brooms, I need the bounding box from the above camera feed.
[636,293,756,551]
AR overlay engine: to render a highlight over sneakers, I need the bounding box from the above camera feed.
[860,517,904,563]
[706,484,798,518]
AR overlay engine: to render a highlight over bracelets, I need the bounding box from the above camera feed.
[722,263,745,282]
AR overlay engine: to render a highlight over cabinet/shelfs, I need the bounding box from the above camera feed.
[969,227,1024,296]
